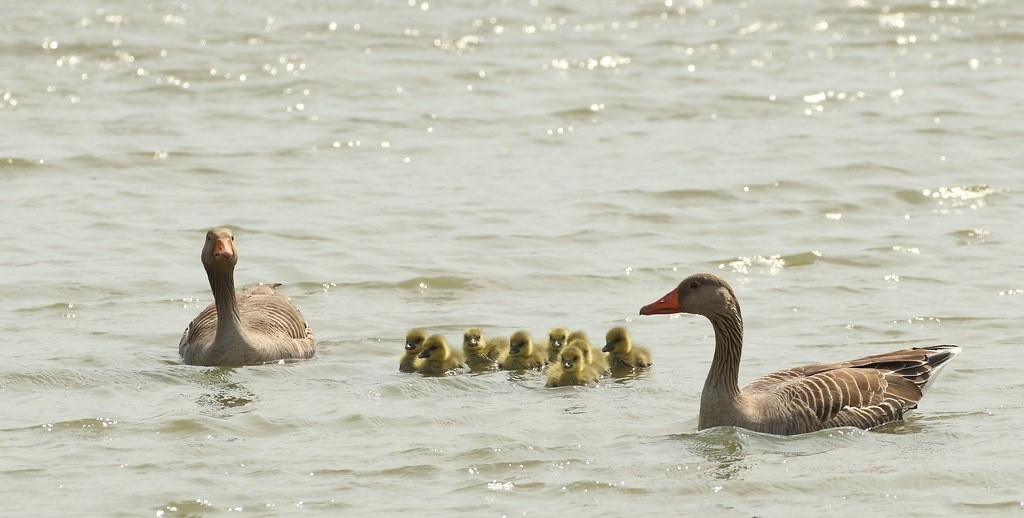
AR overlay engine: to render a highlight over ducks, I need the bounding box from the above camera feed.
[399,325,653,387]
[178,226,317,367]
[639,273,963,437]
